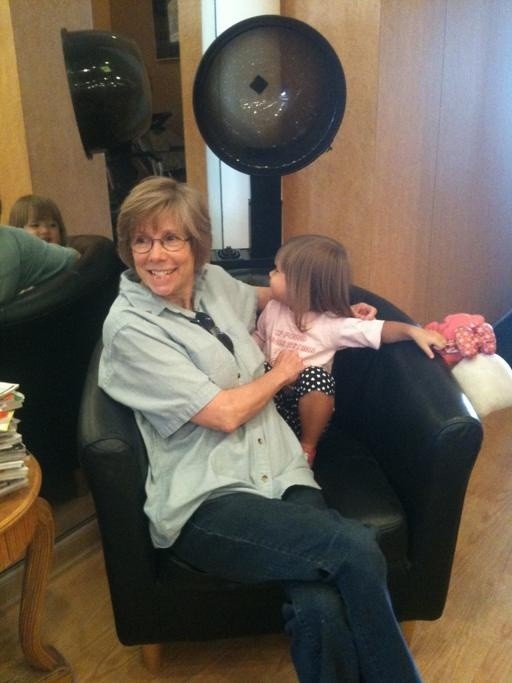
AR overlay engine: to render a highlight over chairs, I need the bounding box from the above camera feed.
[0,235,119,497]
[77,266,484,672]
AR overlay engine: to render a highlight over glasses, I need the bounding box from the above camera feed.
[192,310,233,353]
[131,235,191,254]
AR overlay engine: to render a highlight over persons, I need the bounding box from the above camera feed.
[7,194,69,248]
[0,224,82,304]
[250,234,447,470]
[96,176,423,683]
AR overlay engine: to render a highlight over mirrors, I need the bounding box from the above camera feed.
[0,0,282,552]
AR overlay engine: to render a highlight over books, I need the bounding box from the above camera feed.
[0,381,31,499]
